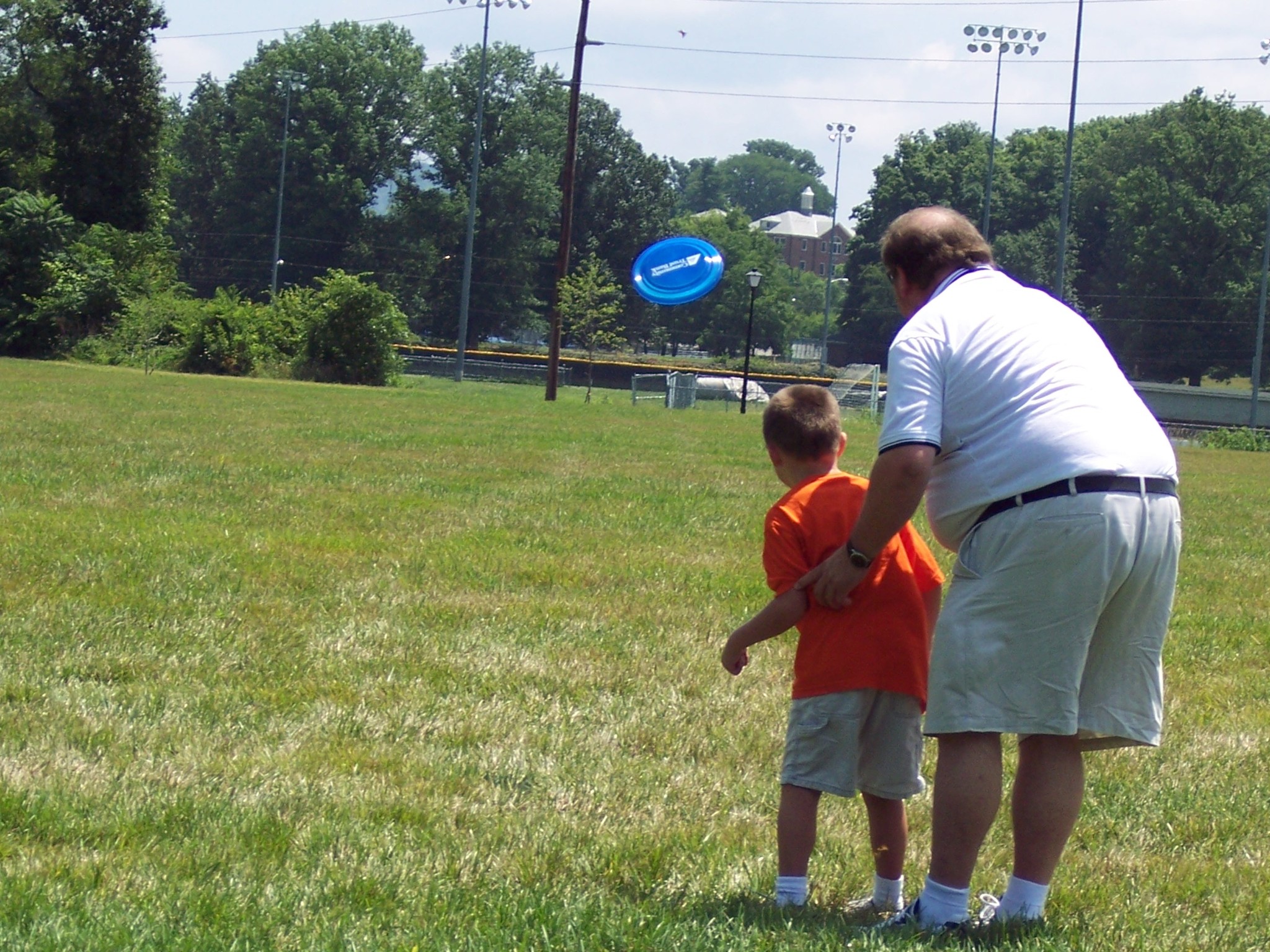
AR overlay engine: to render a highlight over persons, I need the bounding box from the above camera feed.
[718,380,946,926]
[793,205,1184,943]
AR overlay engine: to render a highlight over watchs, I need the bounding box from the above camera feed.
[846,540,875,569]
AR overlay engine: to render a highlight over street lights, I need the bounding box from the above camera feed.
[818,121,858,375]
[1251,38,1270,428]
[266,70,312,301]
[740,267,765,414]
[446,0,532,384]
[963,22,1048,242]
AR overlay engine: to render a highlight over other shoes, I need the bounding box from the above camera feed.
[842,895,902,925]
[872,900,971,933]
[963,895,1041,932]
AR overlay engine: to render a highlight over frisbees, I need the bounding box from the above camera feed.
[631,237,724,305]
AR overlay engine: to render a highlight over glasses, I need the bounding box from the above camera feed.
[886,266,901,284]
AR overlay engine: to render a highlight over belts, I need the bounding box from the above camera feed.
[977,477,1181,525]
